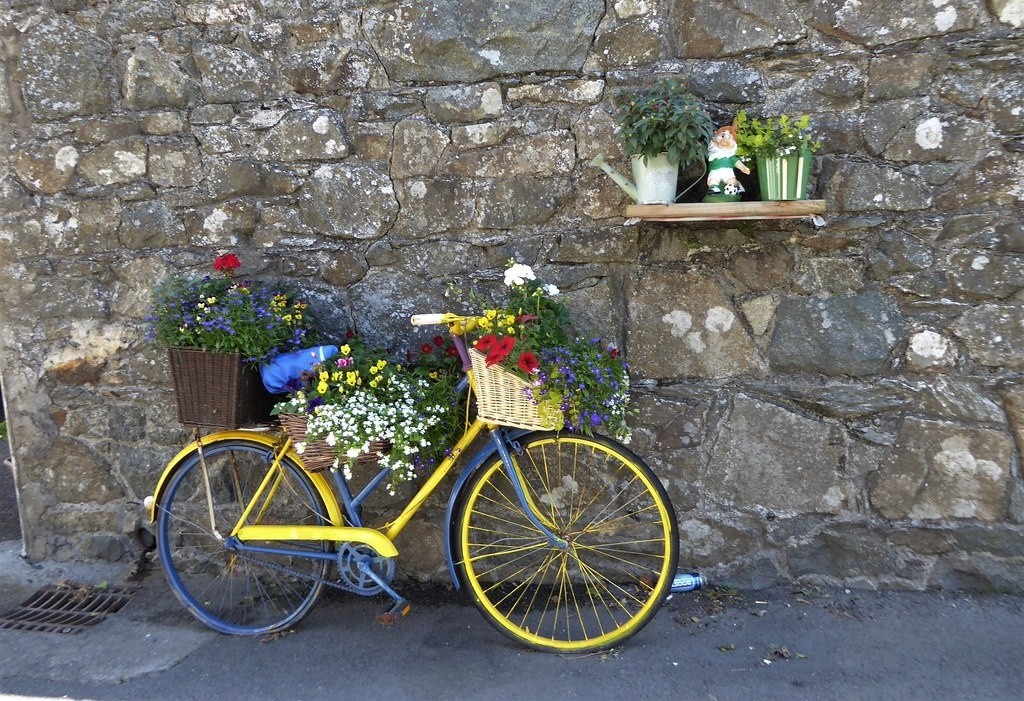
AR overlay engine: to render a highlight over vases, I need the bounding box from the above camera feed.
[757,148,812,202]
[626,151,682,204]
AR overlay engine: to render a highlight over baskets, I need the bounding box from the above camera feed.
[469,348,564,432]
[167,345,267,429]
[277,409,389,472]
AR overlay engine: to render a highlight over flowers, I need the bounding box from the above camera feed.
[737,109,822,171]
[142,253,329,375]
[613,77,713,169]
[445,256,640,445]
[269,331,467,497]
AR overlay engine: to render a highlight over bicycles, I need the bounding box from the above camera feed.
[141,315,681,656]
